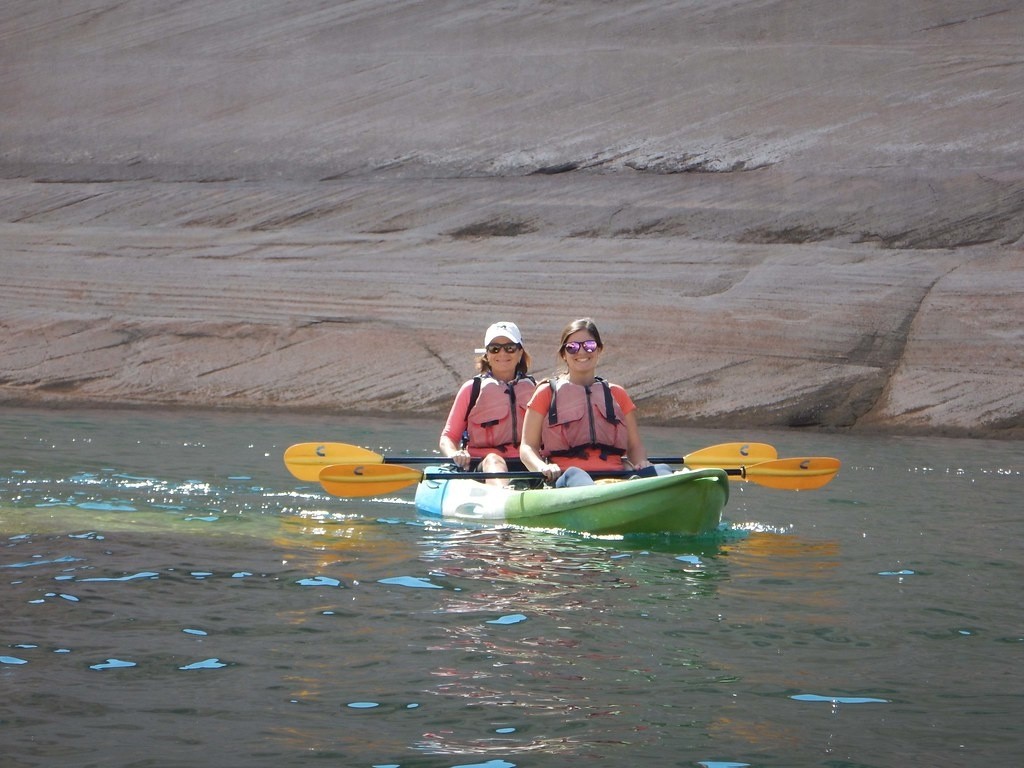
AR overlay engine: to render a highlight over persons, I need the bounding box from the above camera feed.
[520,319,653,487]
[440,322,538,487]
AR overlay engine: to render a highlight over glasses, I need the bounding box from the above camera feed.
[487,343,520,354]
[564,340,599,354]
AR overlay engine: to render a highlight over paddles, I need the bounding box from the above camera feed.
[284,441,777,481]
[318,455,841,498]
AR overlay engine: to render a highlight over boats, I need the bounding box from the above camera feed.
[413,466,731,539]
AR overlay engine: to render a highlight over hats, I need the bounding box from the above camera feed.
[474,322,522,353]
